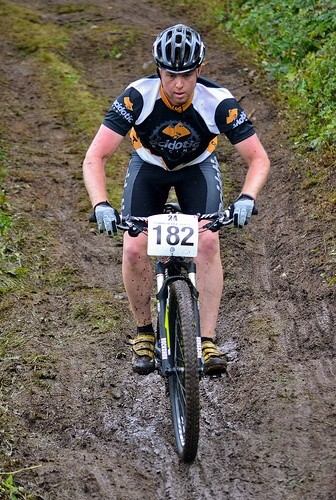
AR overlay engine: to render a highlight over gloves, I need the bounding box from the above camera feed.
[227,194,256,229]
[93,201,120,236]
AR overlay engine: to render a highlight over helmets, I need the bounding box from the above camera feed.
[151,24,207,74]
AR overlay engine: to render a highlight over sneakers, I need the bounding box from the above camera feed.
[126,332,156,375]
[202,341,229,375]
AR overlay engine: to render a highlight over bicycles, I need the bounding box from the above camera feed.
[85,204,260,464]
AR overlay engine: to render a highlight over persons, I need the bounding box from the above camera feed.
[82,20,270,374]
